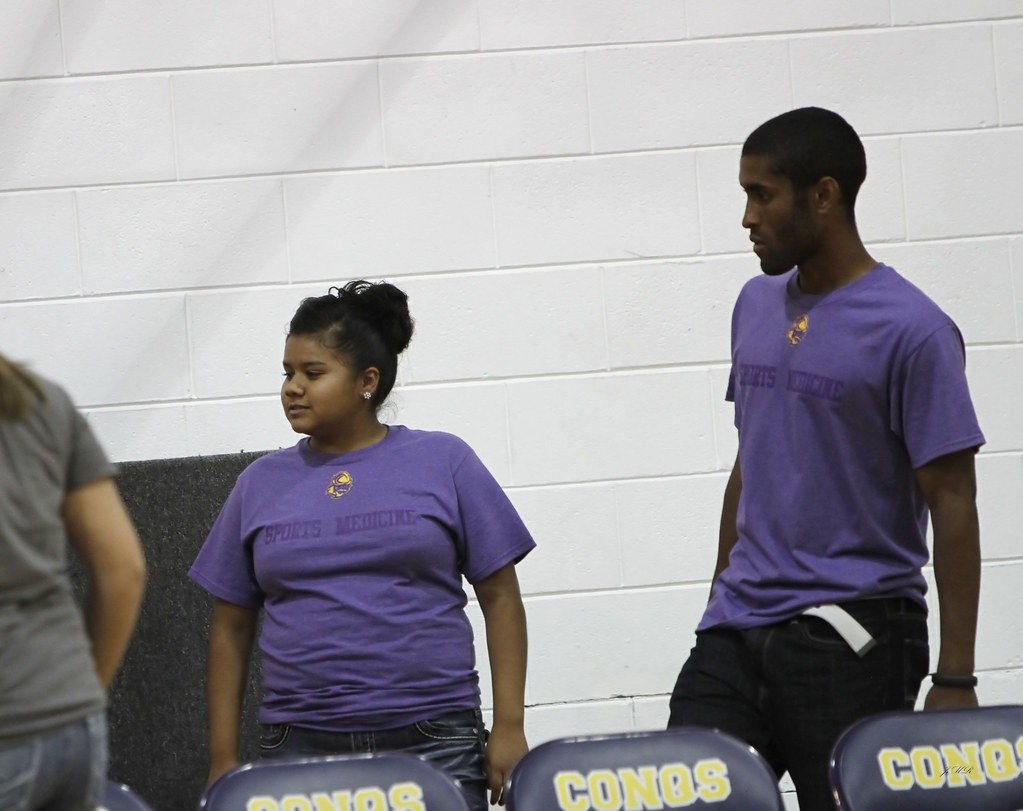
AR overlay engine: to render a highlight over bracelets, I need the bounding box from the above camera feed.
[931,674,978,688]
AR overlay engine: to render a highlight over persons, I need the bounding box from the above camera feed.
[0,346,146,811]
[185,277,538,811]
[666,105,987,811]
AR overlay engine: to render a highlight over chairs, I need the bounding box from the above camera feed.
[502,725,787,811]
[830,700,1023,811]
[203,751,470,811]
